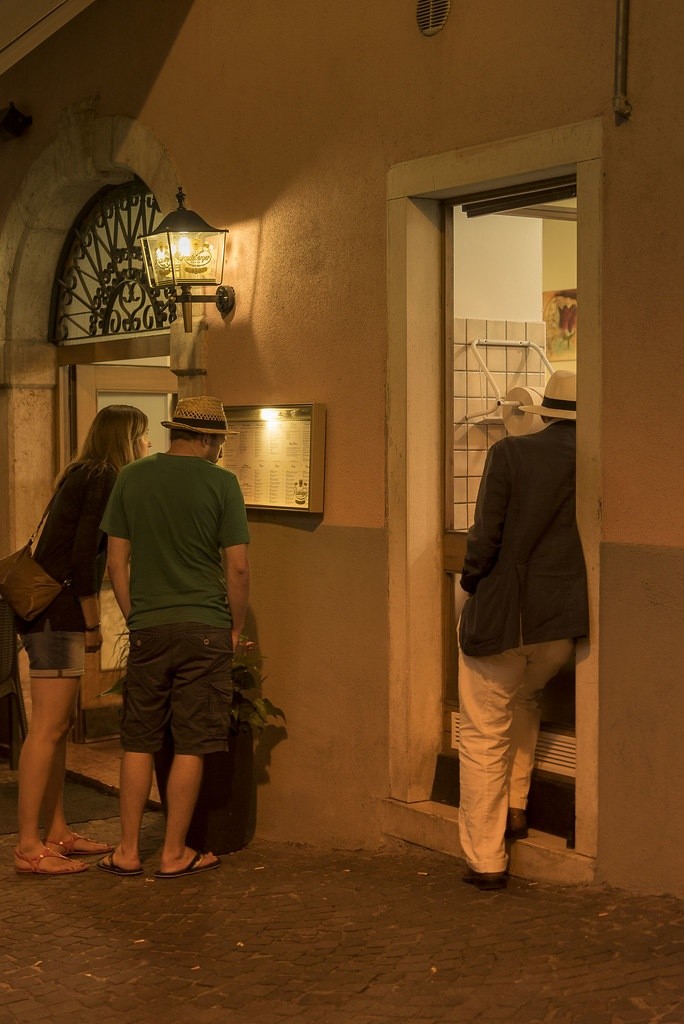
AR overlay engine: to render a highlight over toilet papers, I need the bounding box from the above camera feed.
[502,386,556,436]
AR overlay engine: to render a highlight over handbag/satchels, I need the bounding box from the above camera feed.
[0,545,62,622]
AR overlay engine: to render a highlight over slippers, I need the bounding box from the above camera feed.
[153,848,222,878]
[97,849,143,875]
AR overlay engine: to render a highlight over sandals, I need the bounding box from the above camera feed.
[43,831,115,854]
[14,845,90,874]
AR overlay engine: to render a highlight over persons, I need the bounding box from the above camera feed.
[96,396,251,880]
[13,405,153,875]
[455,369,590,892]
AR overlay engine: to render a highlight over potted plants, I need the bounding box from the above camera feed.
[91,612,285,855]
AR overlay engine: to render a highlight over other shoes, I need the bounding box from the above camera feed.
[461,866,505,890]
[506,808,528,838]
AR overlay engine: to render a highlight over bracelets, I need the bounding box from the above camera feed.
[84,621,101,632]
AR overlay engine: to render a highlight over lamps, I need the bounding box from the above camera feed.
[136,187,235,333]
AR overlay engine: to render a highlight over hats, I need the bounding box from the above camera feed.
[518,370,577,422]
[161,396,240,435]
